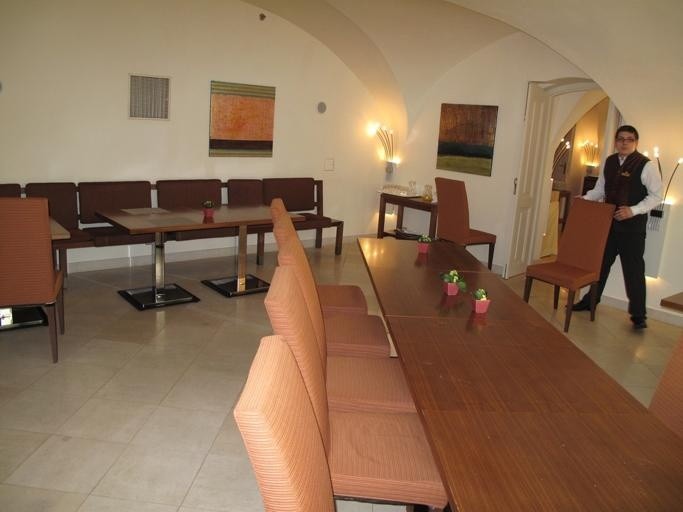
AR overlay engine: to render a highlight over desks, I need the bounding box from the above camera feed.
[356,236,683,511]
[378,193,437,240]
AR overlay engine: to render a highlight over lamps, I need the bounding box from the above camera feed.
[370,120,399,182]
[641,146,683,231]
[579,140,599,176]
[550,138,571,189]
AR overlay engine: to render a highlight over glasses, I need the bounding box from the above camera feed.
[617,138,634,143]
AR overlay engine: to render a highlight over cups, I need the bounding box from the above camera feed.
[423,185,433,201]
[383,184,409,196]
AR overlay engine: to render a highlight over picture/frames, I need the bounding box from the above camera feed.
[126,71,171,121]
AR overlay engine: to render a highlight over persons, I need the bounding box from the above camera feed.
[573,124,664,330]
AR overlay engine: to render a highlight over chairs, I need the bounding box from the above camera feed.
[649,332,683,438]
[523,196,619,333]
[435,176,496,271]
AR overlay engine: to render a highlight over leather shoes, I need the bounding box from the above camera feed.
[633,320,647,329]
[565,301,597,311]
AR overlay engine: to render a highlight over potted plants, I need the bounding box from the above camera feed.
[440,270,465,296]
[470,288,491,313]
